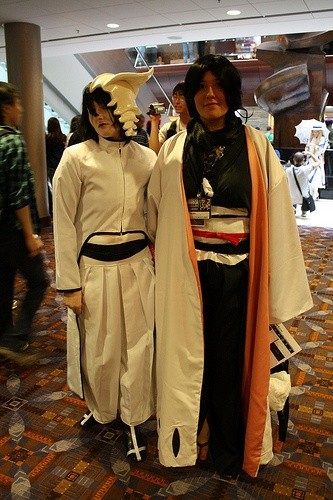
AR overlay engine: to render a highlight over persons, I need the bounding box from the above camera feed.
[148,55,313,475]
[0,80,51,351]
[51,68,158,461]
[45,35,329,219]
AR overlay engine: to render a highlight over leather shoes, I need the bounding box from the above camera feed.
[119,416,151,465]
[78,407,99,429]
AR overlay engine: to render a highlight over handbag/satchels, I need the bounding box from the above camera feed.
[301,195,315,212]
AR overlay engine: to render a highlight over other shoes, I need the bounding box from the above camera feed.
[0,324,38,352]
[194,440,212,467]
[298,211,309,217]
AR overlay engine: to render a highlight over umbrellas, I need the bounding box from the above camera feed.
[294,118,330,145]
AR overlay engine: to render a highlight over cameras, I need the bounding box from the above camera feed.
[149,103,165,115]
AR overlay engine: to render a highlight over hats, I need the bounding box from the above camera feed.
[311,124,323,131]
